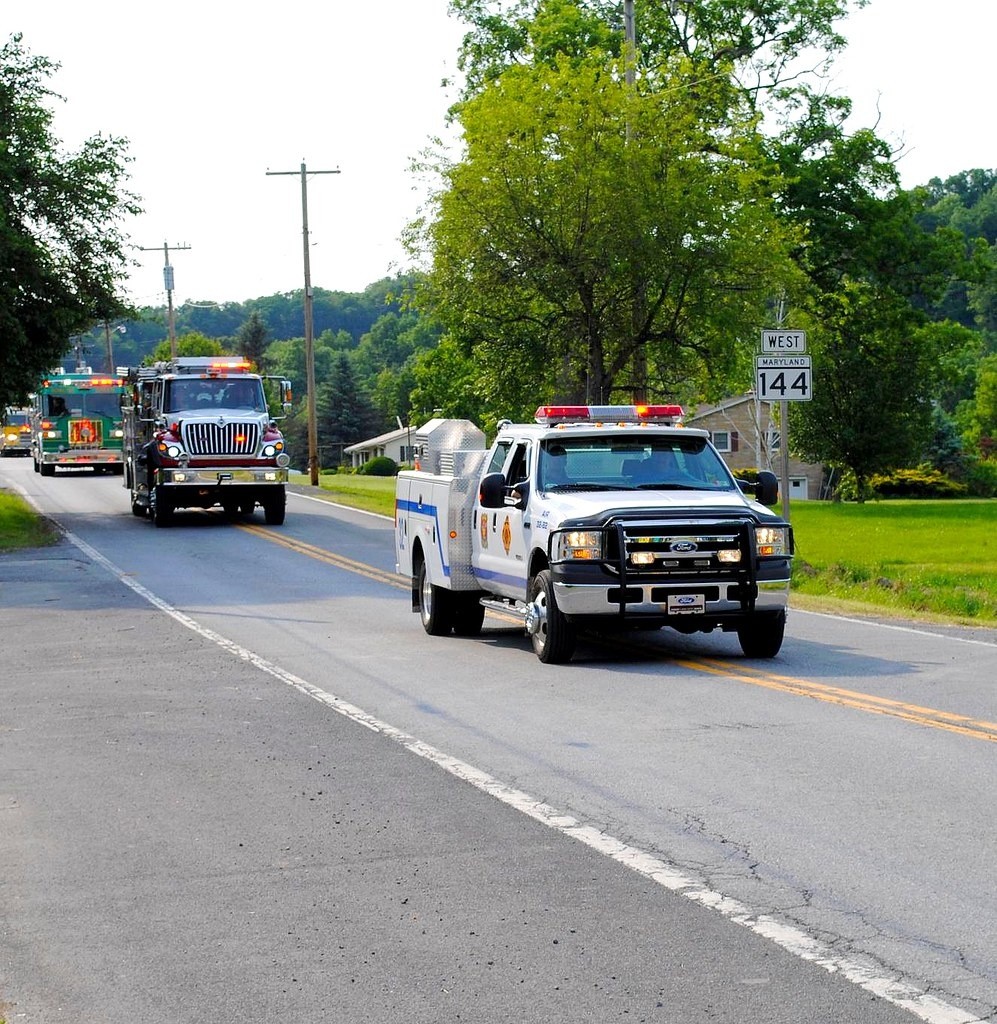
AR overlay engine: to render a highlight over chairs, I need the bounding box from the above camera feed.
[622,459,642,483]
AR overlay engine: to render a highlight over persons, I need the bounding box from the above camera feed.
[47,384,254,414]
[510,445,573,500]
[632,443,708,486]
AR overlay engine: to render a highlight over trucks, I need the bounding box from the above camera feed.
[394,403,793,663]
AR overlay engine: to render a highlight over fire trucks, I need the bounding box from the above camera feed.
[117,355,293,527]
[31,343,126,477]
[0,409,35,456]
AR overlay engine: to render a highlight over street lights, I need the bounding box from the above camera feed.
[104,321,128,373]
